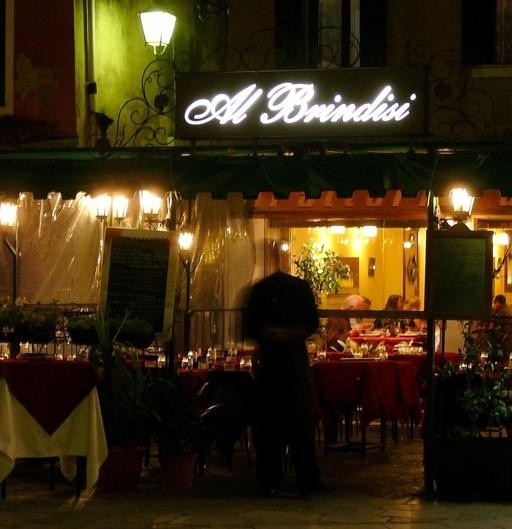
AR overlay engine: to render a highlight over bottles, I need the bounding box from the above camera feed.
[182,346,218,370]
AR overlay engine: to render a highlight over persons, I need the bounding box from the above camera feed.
[491,295,507,334]
[232,239,338,498]
[324,293,422,353]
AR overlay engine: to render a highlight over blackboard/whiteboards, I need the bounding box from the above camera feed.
[426,231,492,321]
[99,228,178,347]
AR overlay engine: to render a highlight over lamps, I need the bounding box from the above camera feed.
[137,0,177,57]
[447,182,474,222]
[90,188,163,225]
[178,200,195,251]
